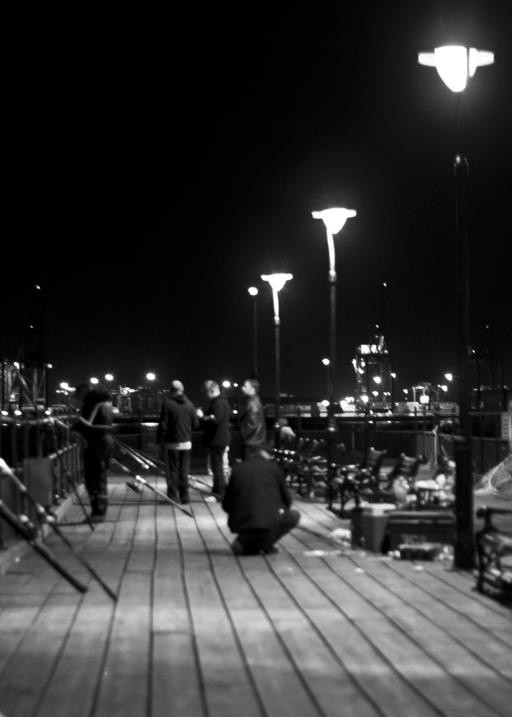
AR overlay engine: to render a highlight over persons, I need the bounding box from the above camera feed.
[278,417,296,436]
[221,442,301,556]
[201,379,232,504]
[68,381,114,523]
[157,378,200,504]
[239,377,268,461]
[207,445,230,476]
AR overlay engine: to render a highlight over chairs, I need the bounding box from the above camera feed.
[272,435,512,598]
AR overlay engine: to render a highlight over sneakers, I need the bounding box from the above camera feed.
[83,515,105,523]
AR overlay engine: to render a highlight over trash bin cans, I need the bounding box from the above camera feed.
[139,421,160,459]
